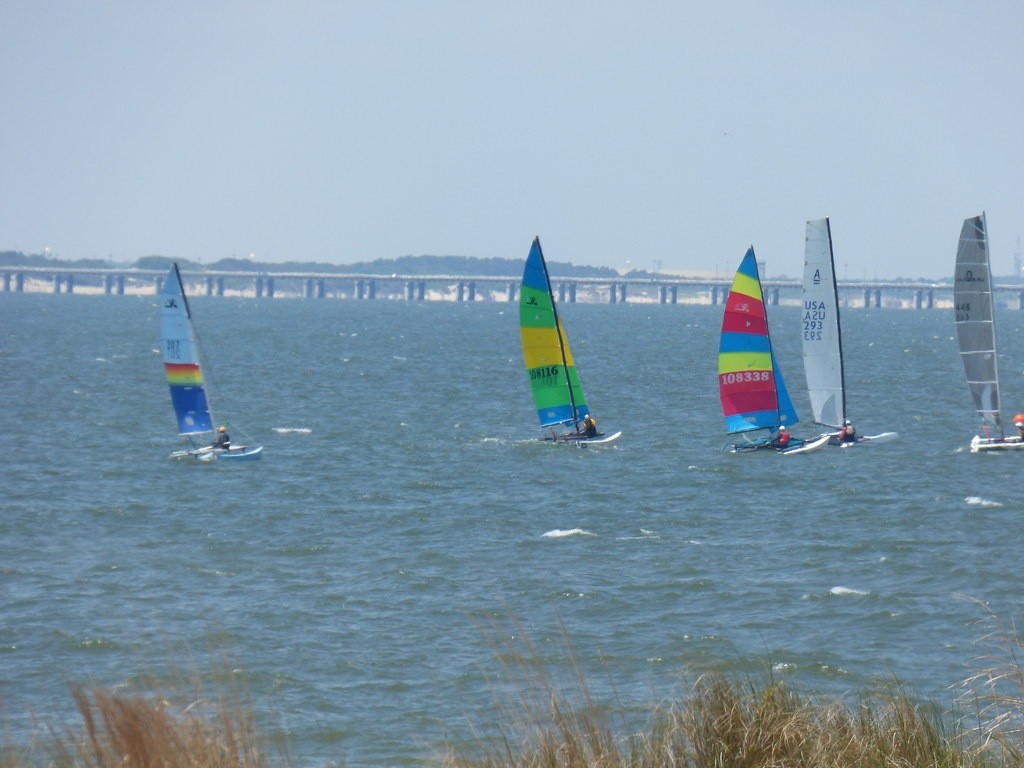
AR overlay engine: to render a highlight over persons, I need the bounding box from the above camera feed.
[779,426,790,446]
[211,426,230,449]
[840,420,855,440]
[1016,422,1024,441]
[583,414,596,436]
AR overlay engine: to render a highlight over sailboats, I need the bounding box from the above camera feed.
[516,238,626,445]
[951,214,1024,450]
[715,244,805,452]
[786,216,896,447]
[158,259,266,462]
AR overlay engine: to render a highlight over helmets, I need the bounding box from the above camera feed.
[585,415,590,420]
[779,426,785,431]
[846,420,850,424]
[219,427,226,432]
